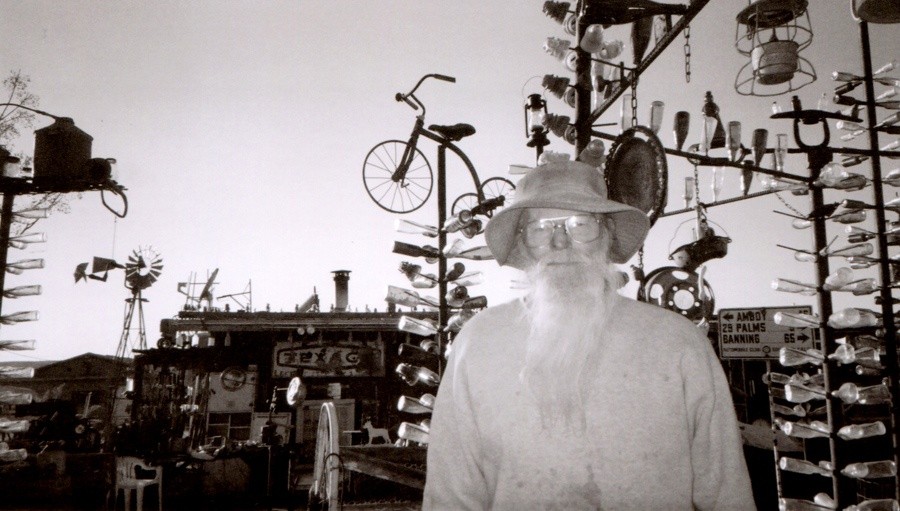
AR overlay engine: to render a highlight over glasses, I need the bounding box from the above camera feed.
[516,213,608,250]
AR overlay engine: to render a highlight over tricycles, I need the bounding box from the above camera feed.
[361,71,517,238]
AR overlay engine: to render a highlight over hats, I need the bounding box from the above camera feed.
[483,162,649,272]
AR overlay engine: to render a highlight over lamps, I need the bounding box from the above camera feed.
[298,324,315,335]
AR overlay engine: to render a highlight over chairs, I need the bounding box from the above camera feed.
[104,456,162,511]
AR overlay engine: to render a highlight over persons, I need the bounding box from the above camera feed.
[421,161,758,511]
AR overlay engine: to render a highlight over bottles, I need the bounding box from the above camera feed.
[0,419,31,432]
[530,29,900,511]
[4,259,45,270]
[0,385,33,405]
[387,196,512,445]
[7,232,50,243]
[0,339,38,351]
[0,449,28,462]
[10,207,50,218]
[0,363,34,379]
[0,284,41,297]
[0,311,39,324]
